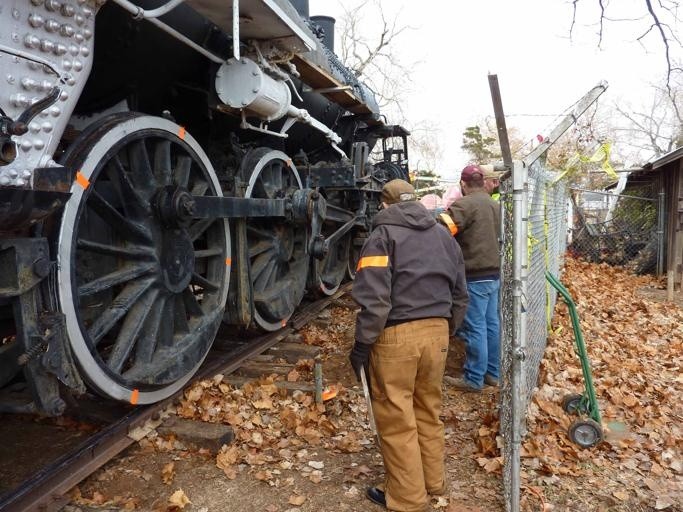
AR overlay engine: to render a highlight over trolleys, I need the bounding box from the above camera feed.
[546,272,627,449]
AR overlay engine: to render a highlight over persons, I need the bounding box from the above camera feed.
[349,179,470,512]
[483,168,503,203]
[435,164,501,393]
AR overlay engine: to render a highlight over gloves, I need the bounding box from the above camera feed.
[349,340,371,382]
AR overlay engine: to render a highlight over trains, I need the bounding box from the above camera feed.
[0,0,412,511]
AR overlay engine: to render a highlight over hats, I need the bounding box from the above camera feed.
[480,164,500,180]
[459,165,483,183]
[378,179,417,210]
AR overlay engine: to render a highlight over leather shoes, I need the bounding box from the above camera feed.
[483,370,498,387]
[441,376,483,393]
[365,486,386,507]
